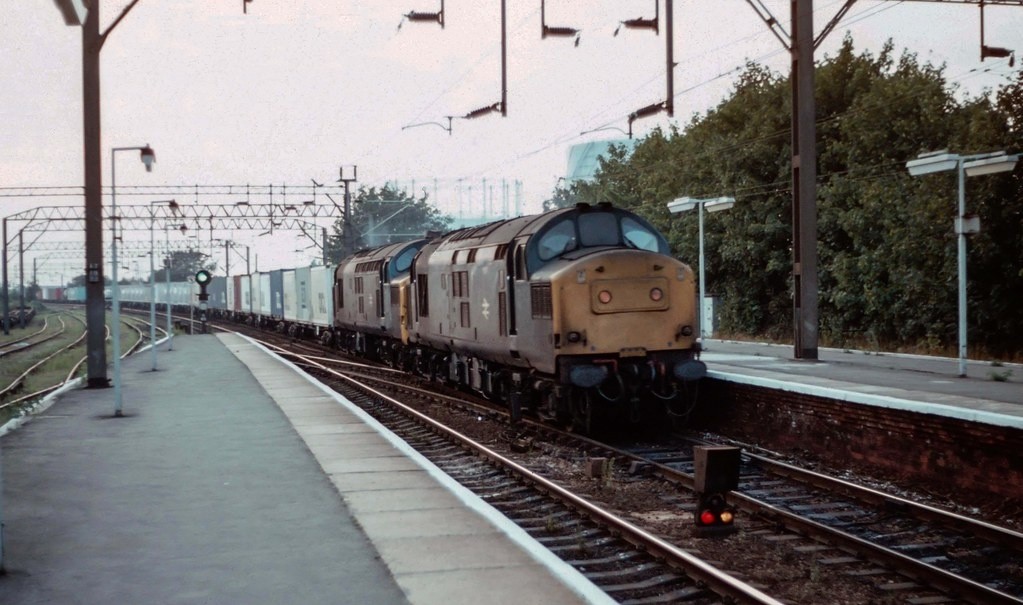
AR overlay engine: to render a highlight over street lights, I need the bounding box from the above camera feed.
[107,142,192,420]
[666,194,737,352]
[902,149,1022,380]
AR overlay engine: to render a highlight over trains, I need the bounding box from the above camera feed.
[29,198,714,436]
